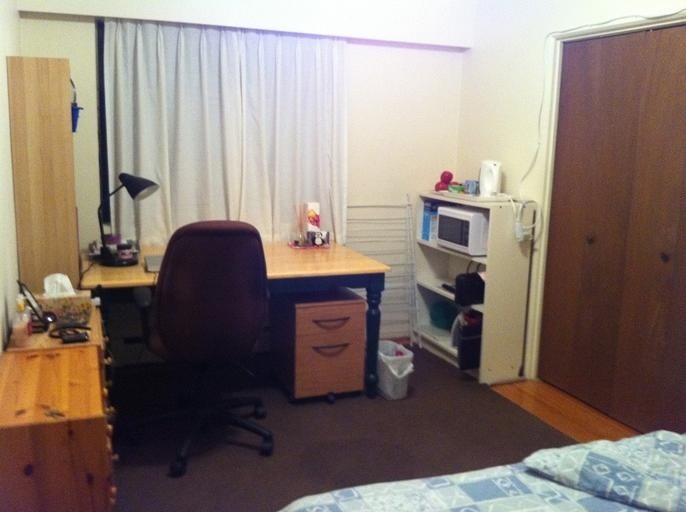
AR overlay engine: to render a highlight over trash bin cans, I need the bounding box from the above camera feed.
[374,339,415,402]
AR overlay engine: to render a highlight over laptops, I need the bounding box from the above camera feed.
[144,254,163,273]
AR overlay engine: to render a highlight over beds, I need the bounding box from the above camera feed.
[277,430,686,512]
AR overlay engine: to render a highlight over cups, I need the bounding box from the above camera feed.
[465,179,480,195]
[289,232,305,248]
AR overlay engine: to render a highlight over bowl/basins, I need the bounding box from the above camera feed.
[447,184,464,193]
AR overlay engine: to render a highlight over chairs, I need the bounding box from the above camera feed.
[133,221,274,477]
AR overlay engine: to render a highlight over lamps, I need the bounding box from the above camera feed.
[98,173,159,267]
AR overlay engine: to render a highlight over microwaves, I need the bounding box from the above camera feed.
[436,206,488,256]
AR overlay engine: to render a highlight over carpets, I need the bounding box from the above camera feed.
[113,345,579,511]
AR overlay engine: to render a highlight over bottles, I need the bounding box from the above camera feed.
[12,296,33,348]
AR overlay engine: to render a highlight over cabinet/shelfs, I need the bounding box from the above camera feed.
[414,192,535,385]
[6,298,113,387]
[280,289,366,403]
[7,57,80,293]
[0,345,119,512]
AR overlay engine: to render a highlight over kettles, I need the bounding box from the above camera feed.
[480,159,504,200]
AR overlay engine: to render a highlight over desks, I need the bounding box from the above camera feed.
[80,241,384,403]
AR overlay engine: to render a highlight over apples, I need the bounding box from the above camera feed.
[435,170,458,191]
